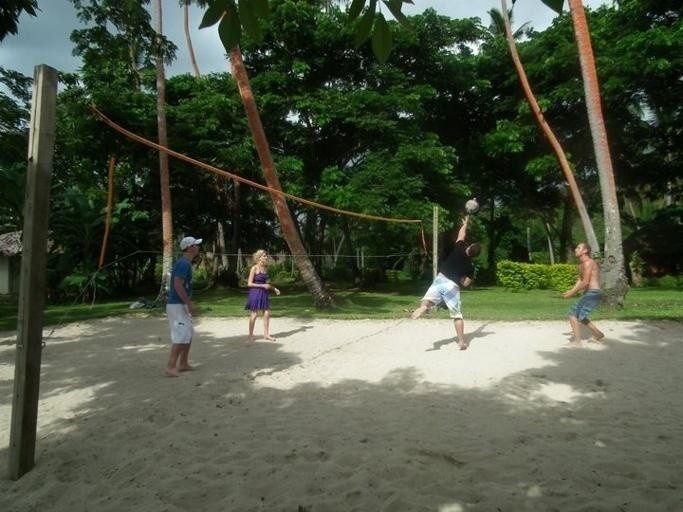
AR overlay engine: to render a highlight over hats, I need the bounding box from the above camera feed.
[180,237,202,251]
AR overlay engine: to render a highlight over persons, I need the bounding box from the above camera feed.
[162,235,204,378]
[412,215,482,350]
[560,241,607,347]
[242,250,282,346]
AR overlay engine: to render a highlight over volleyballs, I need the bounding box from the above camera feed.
[465,200,479,213]
